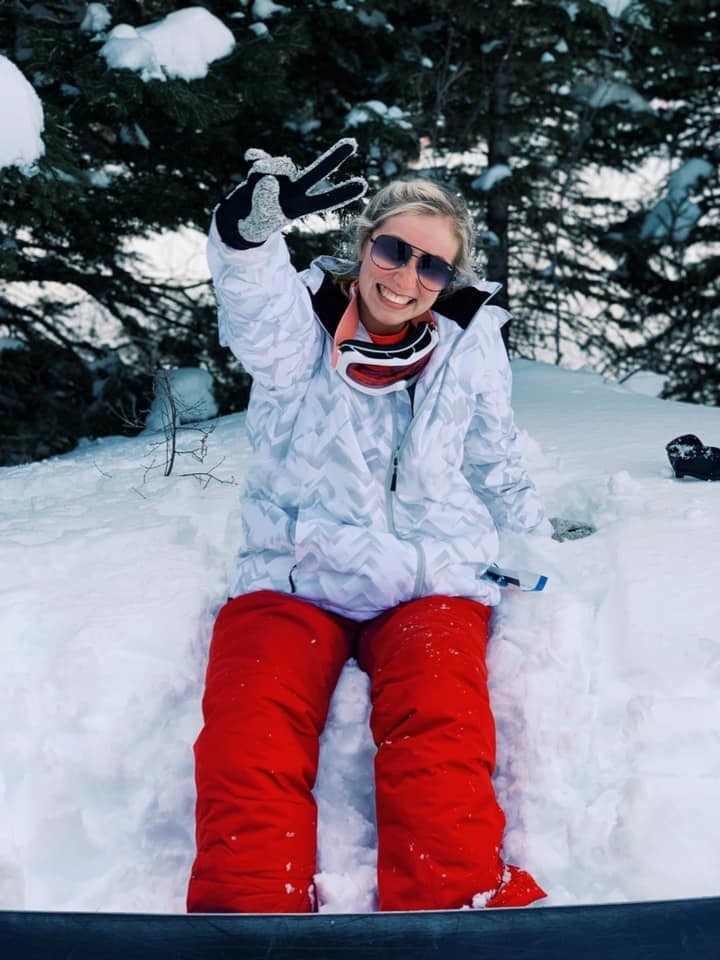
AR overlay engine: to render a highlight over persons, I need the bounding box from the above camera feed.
[186,137,548,916]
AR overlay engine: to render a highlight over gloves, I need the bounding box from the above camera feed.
[550,516,594,543]
[216,137,368,250]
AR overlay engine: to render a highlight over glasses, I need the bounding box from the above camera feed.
[336,323,439,395]
[367,235,458,292]
